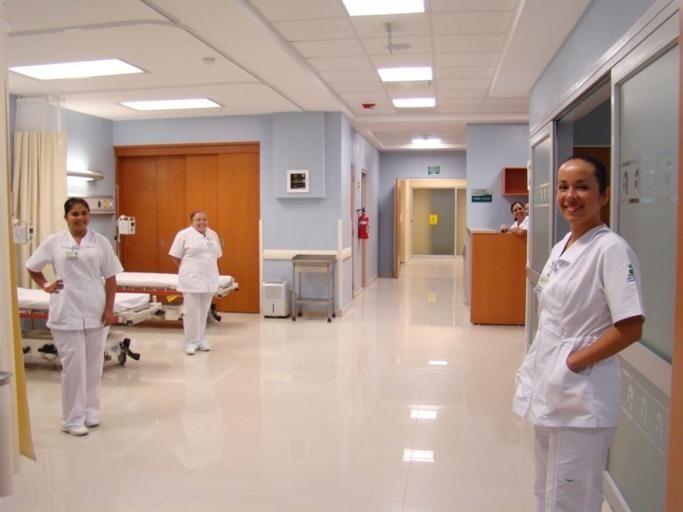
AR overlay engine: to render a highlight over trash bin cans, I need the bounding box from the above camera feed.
[261,280,290,318]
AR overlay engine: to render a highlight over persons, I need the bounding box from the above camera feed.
[524,204,529,216]
[27,197,124,437]
[167,209,223,355]
[512,152,646,512]
[501,200,528,238]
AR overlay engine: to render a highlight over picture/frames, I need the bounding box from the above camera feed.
[286,170,309,193]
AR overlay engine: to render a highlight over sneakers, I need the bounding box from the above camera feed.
[61,425,87,436]
[84,418,100,427]
[198,343,210,351]
[185,347,196,355]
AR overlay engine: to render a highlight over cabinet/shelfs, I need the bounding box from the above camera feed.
[290,253,336,324]
[499,166,529,195]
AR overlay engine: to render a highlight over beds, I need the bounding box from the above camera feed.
[15,284,164,369]
[115,270,239,330]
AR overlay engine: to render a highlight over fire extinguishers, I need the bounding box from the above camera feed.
[356,208,369,239]
